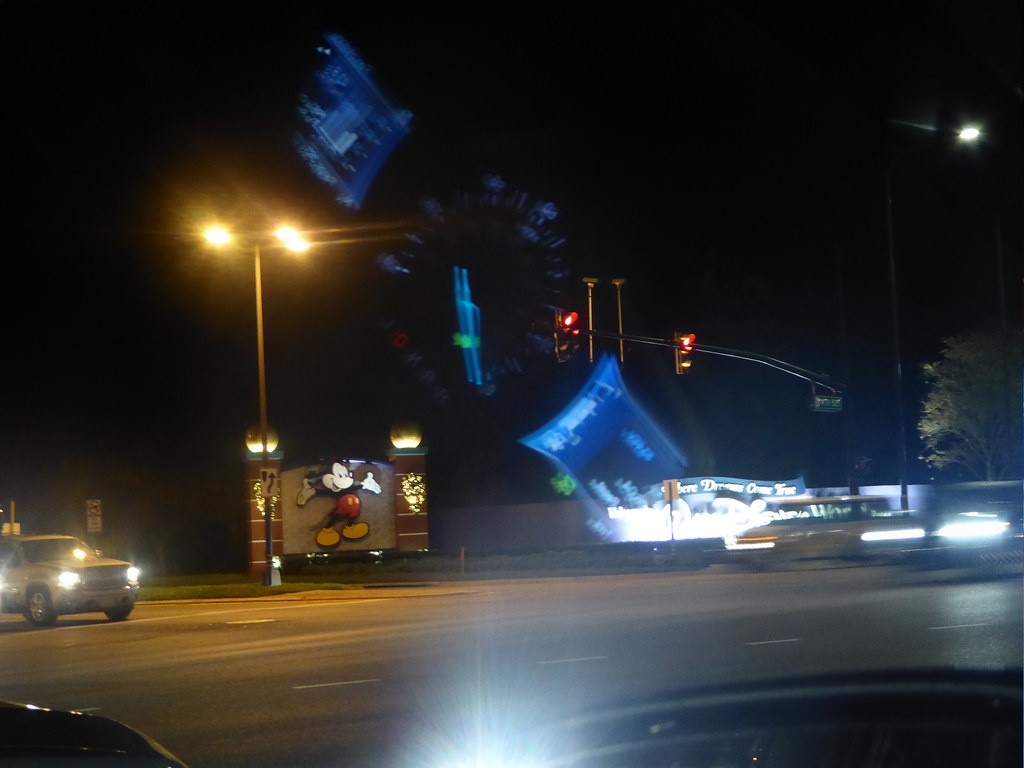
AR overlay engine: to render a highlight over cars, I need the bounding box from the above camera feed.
[728,493,900,570]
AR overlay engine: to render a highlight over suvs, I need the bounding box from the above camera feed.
[0,533,143,628]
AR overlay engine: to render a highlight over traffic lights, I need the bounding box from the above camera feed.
[552,307,584,363]
[674,331,697,375]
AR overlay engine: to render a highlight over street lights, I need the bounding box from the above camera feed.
[198,221,310,589]
[879,115,982,518]
